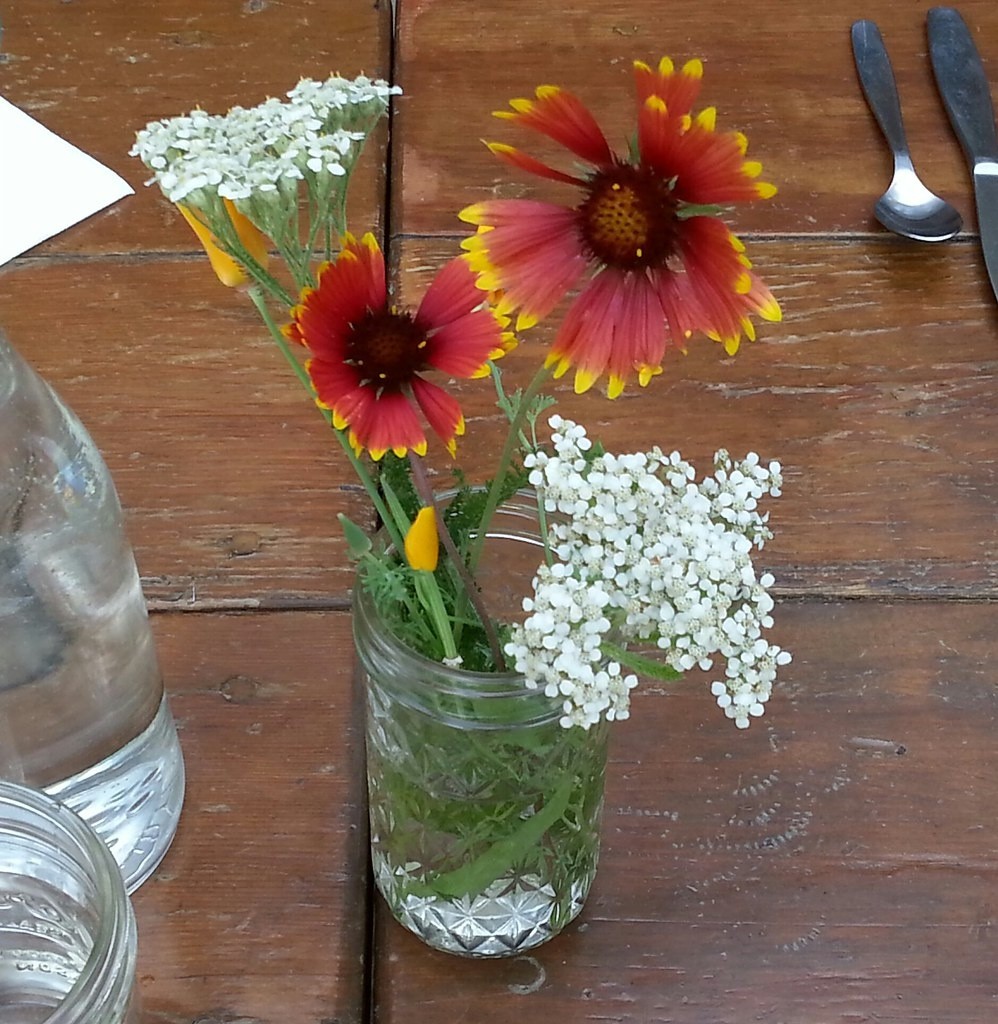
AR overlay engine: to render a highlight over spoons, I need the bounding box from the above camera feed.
[850,17,963,244]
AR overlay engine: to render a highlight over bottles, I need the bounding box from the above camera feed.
[0,340,188,898]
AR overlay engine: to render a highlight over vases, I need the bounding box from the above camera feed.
[352,486,631,957]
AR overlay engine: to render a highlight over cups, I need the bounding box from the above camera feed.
[0,779,142,1024]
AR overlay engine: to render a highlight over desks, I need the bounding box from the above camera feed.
[0,0,998,1024]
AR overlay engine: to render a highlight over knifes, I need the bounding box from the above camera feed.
[925,7,998,302]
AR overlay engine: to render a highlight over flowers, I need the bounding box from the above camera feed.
[128,56,794,922]
